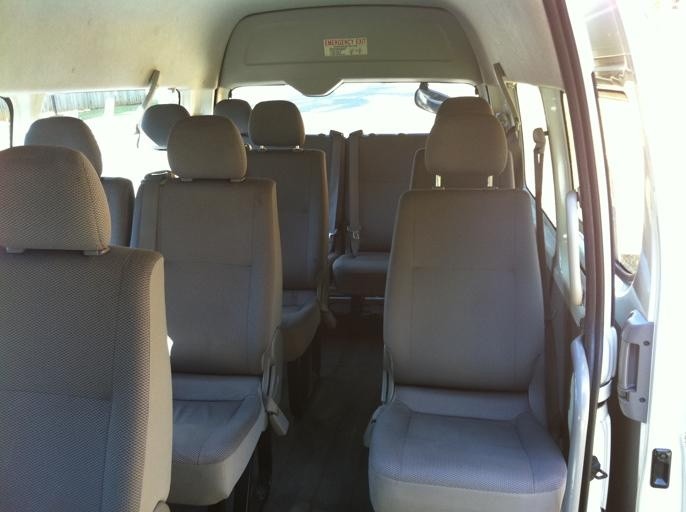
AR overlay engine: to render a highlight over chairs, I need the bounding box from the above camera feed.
[0,96,568,510]
